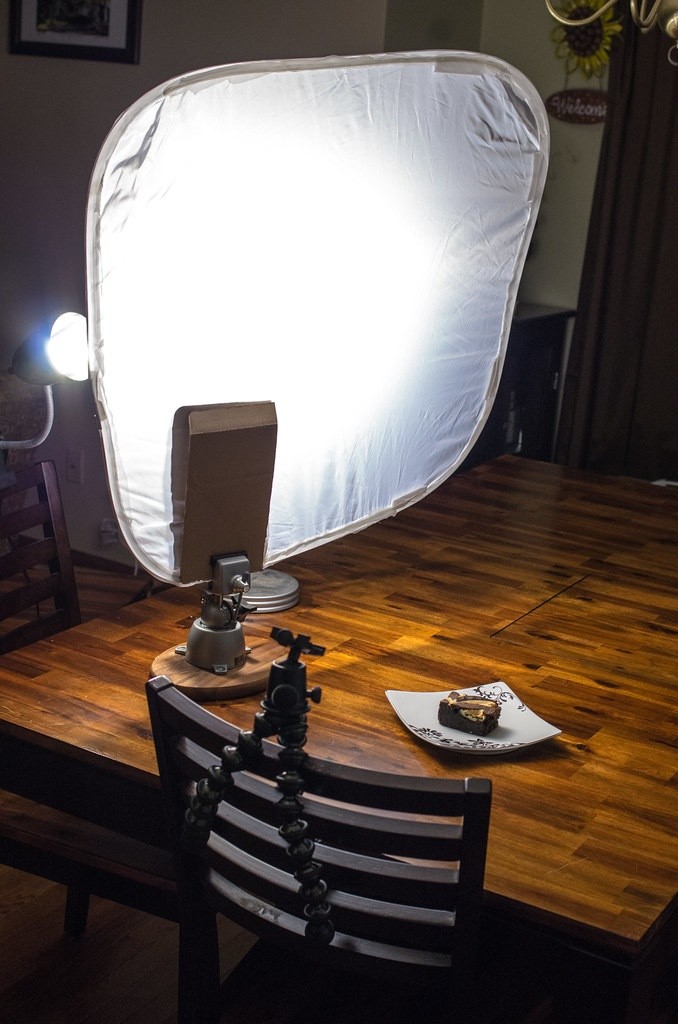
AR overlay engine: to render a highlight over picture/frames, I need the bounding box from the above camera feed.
[3,0,144,68]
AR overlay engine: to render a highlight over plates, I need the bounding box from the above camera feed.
[384,681,564,756]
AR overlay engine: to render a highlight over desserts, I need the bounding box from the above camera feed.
[438,692,502,737]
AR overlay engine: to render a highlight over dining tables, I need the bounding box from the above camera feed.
[0,448,678,1024]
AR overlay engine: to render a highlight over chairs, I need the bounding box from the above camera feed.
[0,459,79,662]
[143,670,494,1024]
